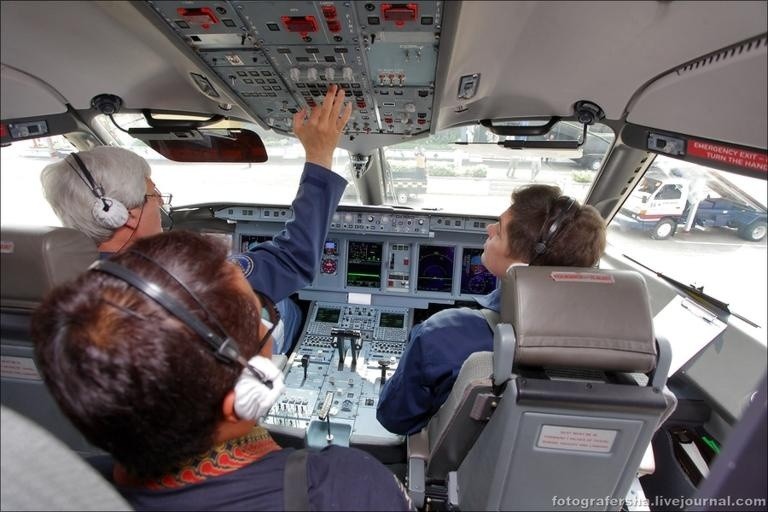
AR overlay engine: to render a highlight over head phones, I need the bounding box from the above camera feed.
[88,249,288,422]
[66,152,130,229]
[502,195,580,276]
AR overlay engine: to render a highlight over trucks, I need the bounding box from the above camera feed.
[453,118,616,171]
[611,164,767,242]
[342,146,427,204]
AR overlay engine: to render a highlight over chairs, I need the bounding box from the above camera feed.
[0,223,112,484]
[406,263,678,512]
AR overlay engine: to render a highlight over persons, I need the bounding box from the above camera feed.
[32,231,418,512]
[376,186,605,434]
[41,85,352,354]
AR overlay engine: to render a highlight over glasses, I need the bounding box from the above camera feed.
[145,194,172,204]
